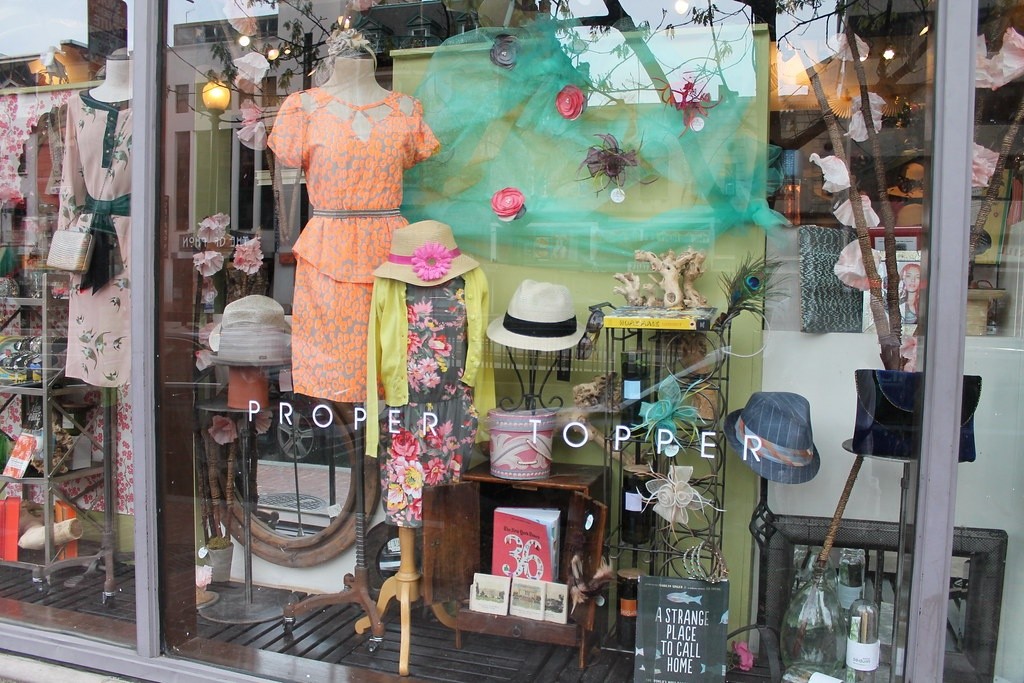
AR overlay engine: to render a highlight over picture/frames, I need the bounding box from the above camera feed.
[865,226,923,252]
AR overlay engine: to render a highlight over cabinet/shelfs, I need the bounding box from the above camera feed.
[589,303,732,653]
[0,274,112,593]
[421,458,611,669]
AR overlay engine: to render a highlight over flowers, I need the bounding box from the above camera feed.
[224,1,269,152]
[191,212,264,302]
[809,24,1024,371]
[412,243,453,282]
[208,409,273,445]
[492,188,525,221]
[490,34,523,68]
[195,323,220,372]
[556,85,586,120]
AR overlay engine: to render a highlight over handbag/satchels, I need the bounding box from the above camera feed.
[47,229,95,274]
[852,369,982,462]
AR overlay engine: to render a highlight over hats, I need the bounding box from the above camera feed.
[370,219,480,286]
[485,279,586,351]
[723,391,821,485]
[209,294,291,368]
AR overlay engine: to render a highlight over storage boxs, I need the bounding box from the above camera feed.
[764,222,864,334]
[604,307,719,330]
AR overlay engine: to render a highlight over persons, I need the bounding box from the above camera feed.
[58,55,131,388]
[269,51,490,576]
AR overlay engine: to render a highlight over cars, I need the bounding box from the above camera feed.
[161,331,360,464]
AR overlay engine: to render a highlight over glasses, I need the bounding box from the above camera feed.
[576,307,606,360]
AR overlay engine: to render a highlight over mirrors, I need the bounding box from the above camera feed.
[758,515,1009,683]
[211,391,381,570]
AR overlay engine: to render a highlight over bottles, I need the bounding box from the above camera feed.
[839,548,864,629]
